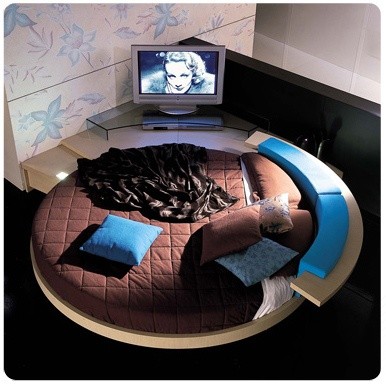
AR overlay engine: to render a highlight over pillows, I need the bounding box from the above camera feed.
[240,151,302,208]
[270,210,312,280]
[80,215,163,265]
[244,193,293,233]
[201,204,262,264]
[215,237,299,287]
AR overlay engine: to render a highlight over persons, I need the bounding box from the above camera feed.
[144,52,215,95]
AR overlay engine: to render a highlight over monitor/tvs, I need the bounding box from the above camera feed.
[131,44,226,116]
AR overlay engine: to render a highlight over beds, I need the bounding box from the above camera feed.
[29,131,364,350]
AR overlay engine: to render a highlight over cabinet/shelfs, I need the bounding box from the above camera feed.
[23,106,344,193]
